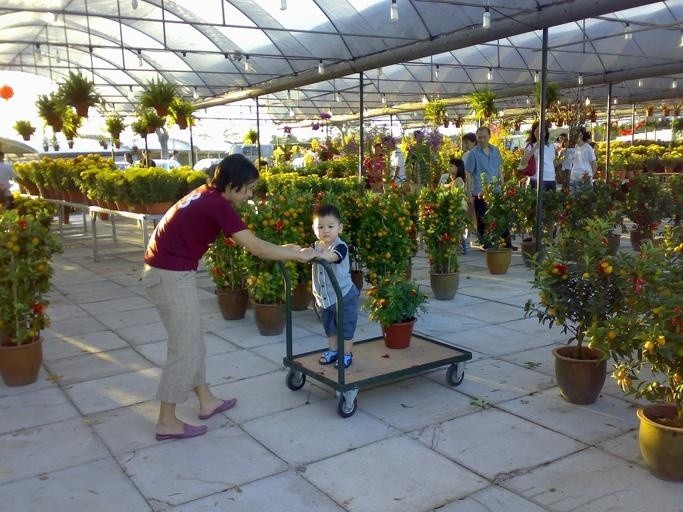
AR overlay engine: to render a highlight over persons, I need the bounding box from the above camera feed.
[382,136,387,146]
[124,152,132,164]
[405,131,431,197]
[524,130,535,151]
[465,127,519,253]
[364,143,387,188]
[463,132,480,244]
[384,137,406,188]
[312,204,360,368]
[555,127,597,194]
[321,143,338,162]
[131,145,140,159]
[143,155,319,440]
[520,120,558,249]
[140,149,156,169]
[0,151,20,203]
[170,150,180,161]
[438,159,464,191]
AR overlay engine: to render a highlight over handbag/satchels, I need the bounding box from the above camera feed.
[519,143,535,176]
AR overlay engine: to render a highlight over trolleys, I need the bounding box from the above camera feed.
[277,257,472,418]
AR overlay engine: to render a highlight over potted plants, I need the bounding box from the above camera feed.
[469,92,496,117]
[285,193,312,312]
[13,121,34,140]
[594,177,621,256]
[102,144,109,150]
[511,115,522,132]
[544,110,554,129]
[523,220,622,405]
[169,98,196,129]
[113,161,128,211]
[245,130,257,144]
[47,160,61,199]
[99,138,105,145]
[520,186,556,267]
[101,157,117,210]
[555,107,564,127]
[140,80,175,116]
[479,172,518,275]
[63,159,82,202]
[144,169,171,214]
[107,117,125,139]
[479,107,483,107]
[534,84,557,109]
[243,208,290,337]
[87,153,97,205]
[321,190,363,297]
[559,179,590,266]
[43,141,49,152]
[361,219,421,349]
[658,100,669,115]
[565,106,578,126]
[624,174,659,251]
[207,235,249,320]
[280,145,293,161]
[32,161,46,197]
[95,154,105,209]
[63,118,81,140]
[13,162,27,193]
[588,107,598,123]
[452,114,463,128]
[61,71,100,117]
[72,155,92,204]
[132,122,148,138]
[426,100,450,128]
[366,190,417,281]
[51,138,59,152]
[38,157,53,198]
[141,108,158,133]
[113,139,121,148]
[642,104,655,118]
[21,163,41,195]
[662,175,683,224]
[68,142,74,148]
[580,108,587,124]
[55,159,66,201]
[123,167,146,213]
[1,209,64,386]
[584,224,683,482]
[419,186,465,301]
[37,94,64,132]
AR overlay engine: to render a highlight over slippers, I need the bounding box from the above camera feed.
[197,398,237,420]
[156,423,207,441]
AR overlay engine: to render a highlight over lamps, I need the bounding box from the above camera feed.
[421,94,428,103]
[245,56,251,72]
[578,73,584,86]
[487,69,494,80]
[435,66,441,78]
[623,23,633,40]
[584,97,591,106]
[390,0,399,21]
[35,45,42,61]
[136,51,144,67]
[679,29,683,48]
[638,79,645,88]
[128,87,134,99]
[381,94,386,104]
[671,78,678,89]
[337,93,342,103]
[193,87,199,100]
[364,109,368,117]
[525,96,532,105]
[132,0,138,9]
[557,98,562,105]
[279,1,288,12]
[319,60,325,75]
[613,98,619,104]
[534,71,540,83]
[482,5,491,29]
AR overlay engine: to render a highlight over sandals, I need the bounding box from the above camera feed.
[318,349,337,365]
[335,352,352,368]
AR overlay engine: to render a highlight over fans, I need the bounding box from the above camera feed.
[253,172,269,207]
